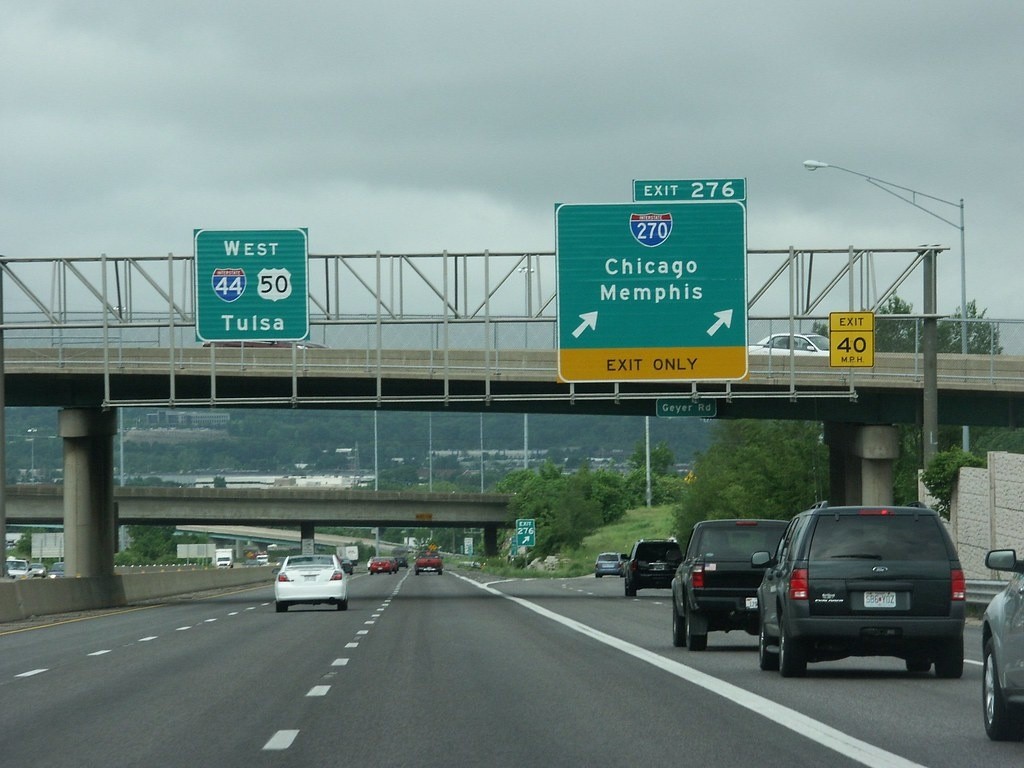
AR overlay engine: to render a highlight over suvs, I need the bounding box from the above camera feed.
[752,500,968,681]
[621,537,685,597]
[665,516,790,652]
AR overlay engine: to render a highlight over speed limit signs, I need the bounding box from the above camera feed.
[257,266,292,301]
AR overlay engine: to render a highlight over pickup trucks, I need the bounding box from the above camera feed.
[414,549,444,575]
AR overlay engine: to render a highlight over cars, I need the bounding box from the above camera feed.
[338,559,354,575]
[747,332,831,356]
[596,551,629,578]
[371,557,394,575]
[29,563,47,578]
[367,555,409,572]
[45,561,65,579]
[274,554,352,614]
[981,548,1024,741]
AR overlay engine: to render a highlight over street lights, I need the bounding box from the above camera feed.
[804,158,969,453]
[27,427,38,483]
[112,305,128,486]
[519,264,534,469]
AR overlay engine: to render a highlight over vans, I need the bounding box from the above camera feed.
[256,554,268,566]
[201,341,326,349]
[5,560,32,580]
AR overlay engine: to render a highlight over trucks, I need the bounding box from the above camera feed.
[215,548,233,569]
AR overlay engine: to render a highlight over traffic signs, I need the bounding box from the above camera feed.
[553,202,750,382]
